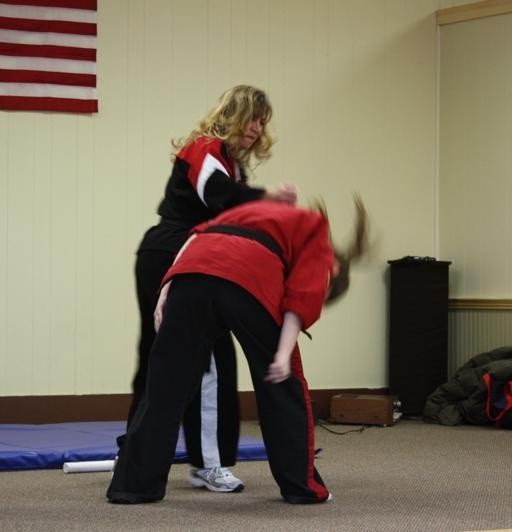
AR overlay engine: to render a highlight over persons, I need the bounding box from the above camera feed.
[104,193,370,504]
[112,84,298,494]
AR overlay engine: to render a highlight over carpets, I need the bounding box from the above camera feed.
[0,420,268,467]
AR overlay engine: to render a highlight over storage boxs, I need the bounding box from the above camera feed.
[329,392,394,427]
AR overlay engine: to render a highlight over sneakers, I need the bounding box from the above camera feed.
[189,466,244,492]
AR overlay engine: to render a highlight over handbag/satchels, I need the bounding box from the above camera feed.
[481,374,511,429]
